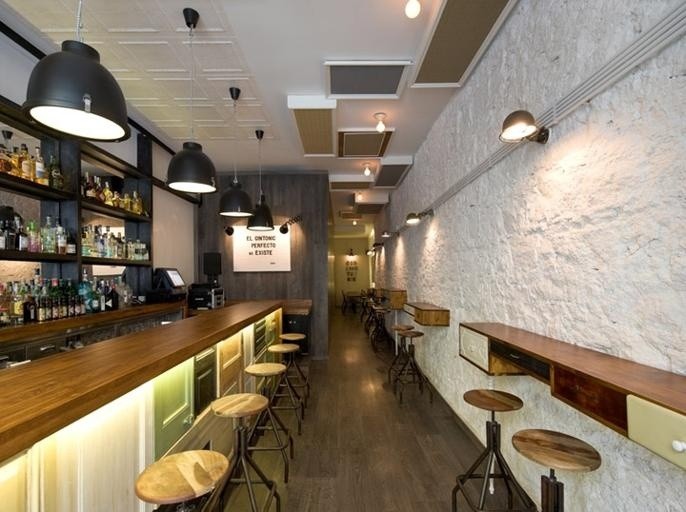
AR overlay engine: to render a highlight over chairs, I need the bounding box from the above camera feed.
[342,290,356,314]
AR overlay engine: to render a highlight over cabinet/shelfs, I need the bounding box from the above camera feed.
[382,290,408,310]
[79,143,152,314]
[367,288,381,297]
[0,95,80,329]
[402,302,450,326]
[458,322,686,472]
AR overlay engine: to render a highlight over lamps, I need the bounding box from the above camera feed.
[20,1,131,144]
[166,7,219,197]
[360,164,375,180]
[349,249,354,257]
[373,242,384,247]
[366,250,376,256]
[401,1,424,21]
[406,208,433,225]
[499,110,550,144]
[374,117,388,136]
[357,192,374,204]
[381,231,399,238]
[346,251,358,263]
[247,129,274,231]
[218,87,255,217]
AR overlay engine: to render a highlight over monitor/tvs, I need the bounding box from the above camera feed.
[163,269,186,288]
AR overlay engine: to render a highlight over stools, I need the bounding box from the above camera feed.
[512,428,602,512]
[277,333,310,408]
[211,393,281,511]
[394,331,433,404]
[451,389,524,512]
[261,344,305,436]
[244,362,294,483]
[388,324,416,384]
[134,449,229,505]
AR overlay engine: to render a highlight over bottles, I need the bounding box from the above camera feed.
[83,271,94,315]
[114,231,127,258]
[52,154,67,190]
[18,142,35,179]
[84,171,98,201]
[38,279,46,322]
[16,217,29,248]
[61,279,67,320]
[79,224,87,256]
[97,224,104,257]
[66,226,78,254]
[97,275,106,313]
[133,190,144,215]
[88,224,93,256]
[8,282,22,323]
[135,235,146,259]
[123,278,137,311]
[1,129,19,177]
[30,220,38,255]
[60,223,67,254]
[43,216,56,255]
[119,277,126,308]
[46,279,56,320]
[106,280,114,313]
[107,227,113,254]
[4,220,16,249]
[50,277,60,320]
[1,224,10,252]
[129,231,136,259]
[104,182,116,207]
[66,278,76,317]
[122,192,143,214]
[36,148,49,185]
[23,285,37,320]
